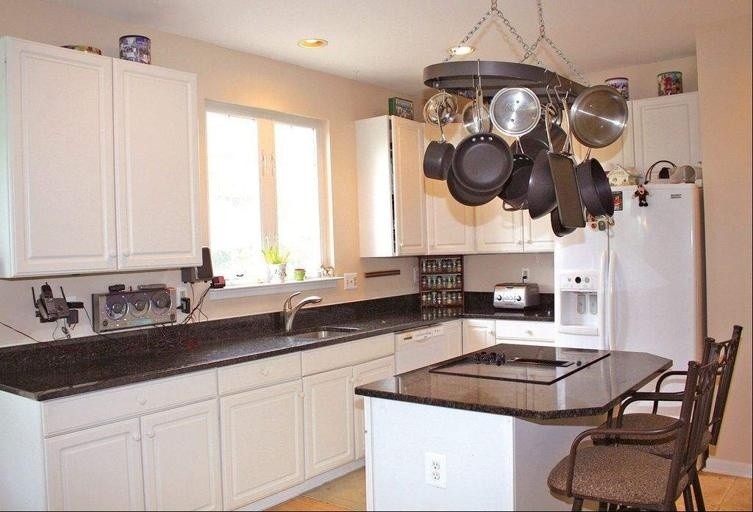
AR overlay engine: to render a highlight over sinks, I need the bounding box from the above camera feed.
[276,326,362,340]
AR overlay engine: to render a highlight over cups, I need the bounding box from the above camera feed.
[294,268,305,281]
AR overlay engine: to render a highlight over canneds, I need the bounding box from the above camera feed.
[657,72,682,96]
[604,77,628,104]
[119,35,150,64]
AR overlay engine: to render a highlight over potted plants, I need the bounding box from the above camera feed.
[260,235,287,283]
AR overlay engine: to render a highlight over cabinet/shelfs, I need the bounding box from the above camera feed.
[462,319,497,355]
[0,36,204,281]
[1,368,221,512]
[301,332,396,486]
[497,319,556,343]
[580,90,701,182]
[219,351,304,512]
[354,117,426,259]
[423,120,474,256]
[473,103,580,255]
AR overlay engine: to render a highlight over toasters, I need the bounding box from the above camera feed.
[491,282,541,310]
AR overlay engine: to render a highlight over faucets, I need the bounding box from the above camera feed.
[283,291,323,330]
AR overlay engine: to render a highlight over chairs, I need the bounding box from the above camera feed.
[547,343,723,511]
[591,325,745,512]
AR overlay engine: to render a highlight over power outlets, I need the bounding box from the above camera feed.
[177,288,189,316]
[423,451,447,489]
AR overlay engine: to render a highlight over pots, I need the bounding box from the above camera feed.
[417,82,630,238]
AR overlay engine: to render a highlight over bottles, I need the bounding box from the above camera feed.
[421,259,462,308]
[421,307,462,320]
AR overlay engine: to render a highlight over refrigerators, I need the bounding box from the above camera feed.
[553,182,703,431]
